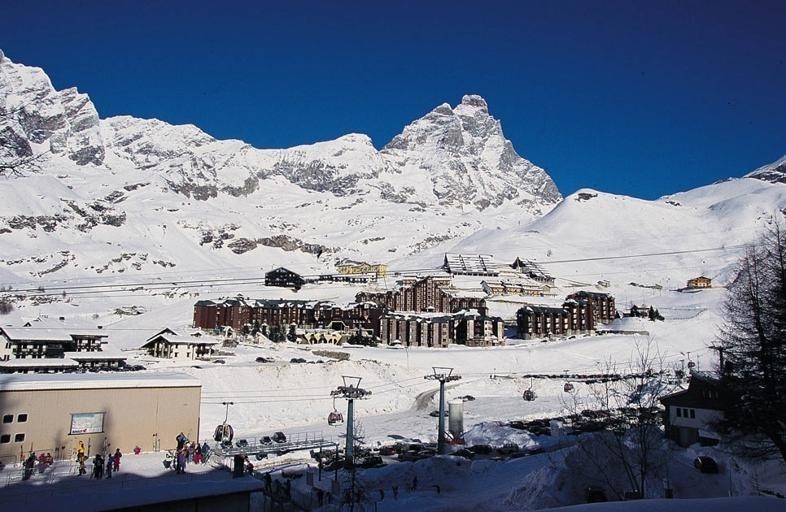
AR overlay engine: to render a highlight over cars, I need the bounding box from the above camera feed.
[448,443,545,462]
[510,405,666,436]
[584,486,608,503]
[62,363,148,374]
[694,455,719,474]
[310,442,439,473]
[256,356,326,364]
[220,431,291,460]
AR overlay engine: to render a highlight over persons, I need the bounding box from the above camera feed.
[275,477,297,501]
[412,475,418,493]
[74,435,122,481]
[378,486,385,501]
[247,461,255,477]
[134,446,141,455]
[264,472,272,494]
[23,451,54,480]
[317,489,324,507]
[392,483,399,498]
[172,430,211,475]
